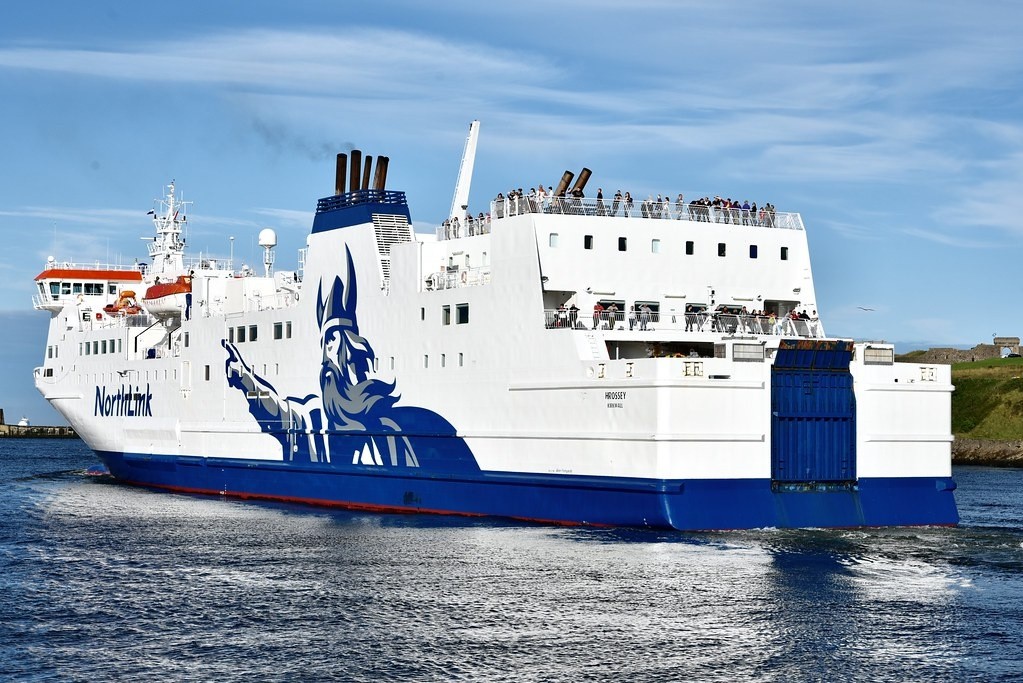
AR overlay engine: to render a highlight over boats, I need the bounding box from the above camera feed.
[141,278,191,317]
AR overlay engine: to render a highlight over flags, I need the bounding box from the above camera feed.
[146,208,154,215]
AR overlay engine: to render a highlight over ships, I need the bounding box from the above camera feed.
[30,118,962,532]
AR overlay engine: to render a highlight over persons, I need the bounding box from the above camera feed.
[494,185,554,218]
[476,212,491,234]
[442,217,460,240]
[675,193,684,220]
[610,190,632,218]
[640,194,670,219]
[464,213,475,236]
[556,187,585,214]
[554,304,568,329]
[607,302,619,330]
[687,196,776,229]
[597,187,604,217]
[684,304,707,332]
[592,301,603,329]
[639,303,652,331]
[781,309,819,338]
[629,305,637,330]
[713,306,775,335]
[569,304,580,330]
